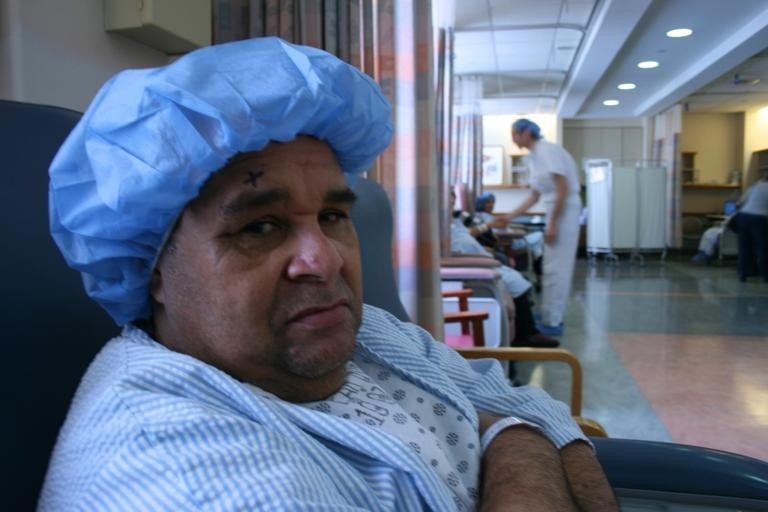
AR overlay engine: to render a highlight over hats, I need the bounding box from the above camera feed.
[516,119,539,136]
[49,36,393,327]
[476,192,495,212]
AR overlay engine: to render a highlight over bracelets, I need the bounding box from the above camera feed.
[477,223,490,234]
[480,414,546,466]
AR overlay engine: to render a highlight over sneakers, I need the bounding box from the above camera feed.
[691,250,715,266]
[738,272,768,283]
[512,322,563,347]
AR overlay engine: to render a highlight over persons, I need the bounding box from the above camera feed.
[690,212,738,267]
[469,190,545,292]
[30,34,628,510]
[493,118,584,337]
[445,185,561,349]
[732,161,768,282]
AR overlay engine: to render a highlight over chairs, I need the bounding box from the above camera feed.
[1,97,768,512]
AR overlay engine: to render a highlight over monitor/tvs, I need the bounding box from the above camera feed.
[723,201,737,216]
